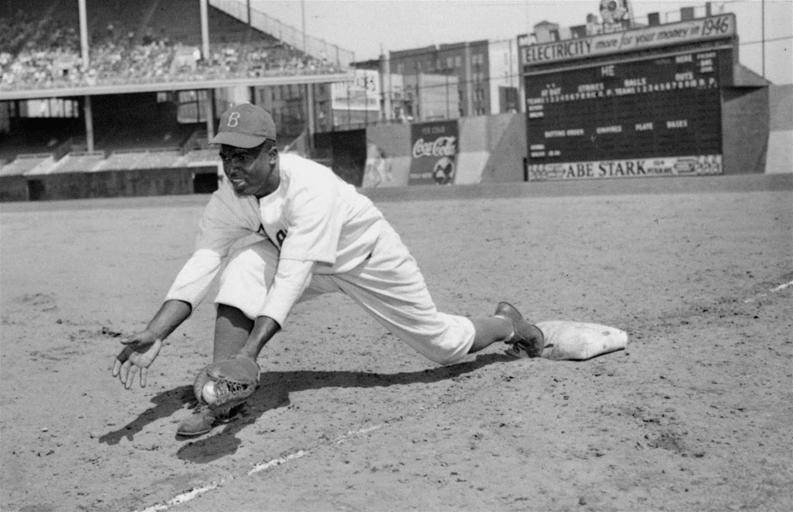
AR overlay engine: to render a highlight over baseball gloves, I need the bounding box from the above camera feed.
[193,354,260,417]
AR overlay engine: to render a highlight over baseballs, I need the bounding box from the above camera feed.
[202,380,218,404]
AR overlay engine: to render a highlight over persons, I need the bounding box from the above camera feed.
[586,14,604,37]
[394,84,415,122]
[113,104,544,436]
[476,89,486,115]
[0,7,335,89]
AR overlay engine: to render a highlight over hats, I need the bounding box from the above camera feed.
[208,103,277,149]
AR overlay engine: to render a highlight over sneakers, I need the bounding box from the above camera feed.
[176,397,251,437]
[494,301,544,358]
